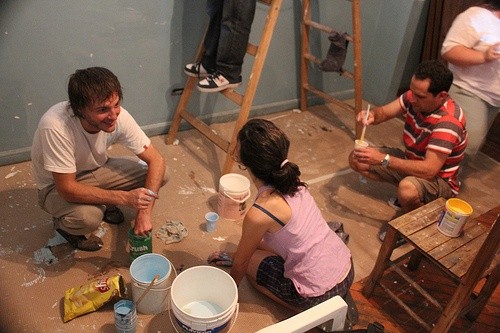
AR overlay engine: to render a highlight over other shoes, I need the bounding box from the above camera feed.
[56,228,103,251]
[379,230,404,243]
[388,197,402,210]
[103,205,125,224]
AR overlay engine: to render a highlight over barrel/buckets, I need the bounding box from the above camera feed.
[168,266,239,333]
[436,198,473,238]
[128,226,153,267]
[217,173,250,222]
[130,253,177,315]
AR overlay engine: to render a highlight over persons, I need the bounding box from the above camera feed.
[440,0,500,157]
[183,0,256,93]
[348,61,469,245]
[31,67,169,251]
[208,119,360,333]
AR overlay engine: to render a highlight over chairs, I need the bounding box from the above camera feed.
[361,198,500,333]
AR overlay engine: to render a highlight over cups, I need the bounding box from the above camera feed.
[205,212,219,232]
[114,299,137,333]
[354,139,368,152]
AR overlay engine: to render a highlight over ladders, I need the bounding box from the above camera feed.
[166,0,362,175]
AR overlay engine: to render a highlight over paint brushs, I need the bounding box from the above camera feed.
[359,104,370,144]
[130,274,160,312]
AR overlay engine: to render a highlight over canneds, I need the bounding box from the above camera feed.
[436,198,473,237]
[128,229,153,263]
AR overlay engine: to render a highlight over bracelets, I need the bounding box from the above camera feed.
[383,154,390,167]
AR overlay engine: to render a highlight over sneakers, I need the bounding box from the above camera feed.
[197,72,240,93]
[184,64,216,77]
